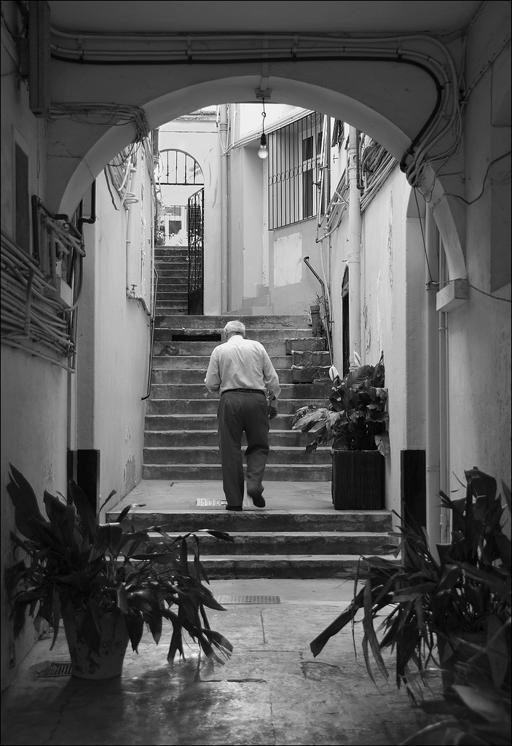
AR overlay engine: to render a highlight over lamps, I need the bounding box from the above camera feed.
[257,96,269,160]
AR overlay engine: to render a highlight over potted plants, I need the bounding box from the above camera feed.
[307,465,512,702]
[5,459,238,683]
[290,350,390,510]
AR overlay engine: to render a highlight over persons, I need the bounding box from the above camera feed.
[203,319,282,509]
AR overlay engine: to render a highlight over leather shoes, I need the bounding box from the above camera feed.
[225,486,266,511]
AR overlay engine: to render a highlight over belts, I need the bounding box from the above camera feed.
[224,388,267,396]
